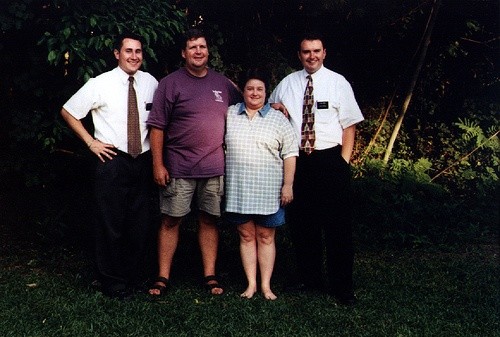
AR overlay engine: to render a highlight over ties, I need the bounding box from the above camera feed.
[127,77,142,159]
[301,75,316,156]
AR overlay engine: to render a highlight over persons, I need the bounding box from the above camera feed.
[60,35,158,299]
[221,73,300,301]
[146,27,290,298]
[269,32,365,305]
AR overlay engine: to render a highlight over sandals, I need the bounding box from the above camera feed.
[146,276,175,300]
[203,275,224,297]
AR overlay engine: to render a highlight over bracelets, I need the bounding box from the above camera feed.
[87,138,95,147]
